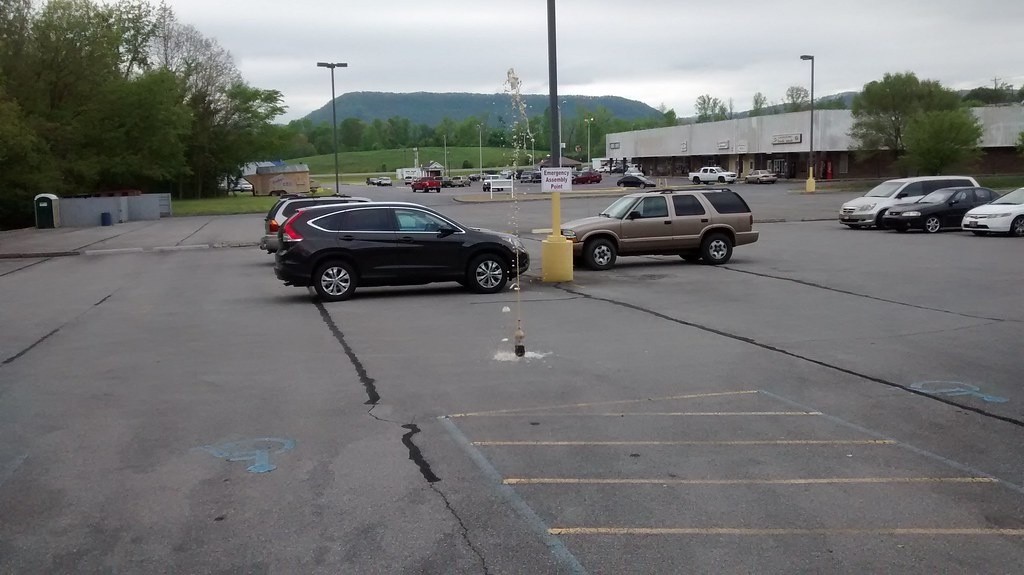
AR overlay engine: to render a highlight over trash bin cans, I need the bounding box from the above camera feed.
[102,212,110,226]
[34,194,61,230]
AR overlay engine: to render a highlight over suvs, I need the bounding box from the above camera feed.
[260,194,379,254]
[566,186,762,271]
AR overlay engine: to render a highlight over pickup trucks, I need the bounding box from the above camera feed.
[688,167,738,185]
[411,177,440,193]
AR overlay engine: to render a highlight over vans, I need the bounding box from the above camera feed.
[404,175,416,185]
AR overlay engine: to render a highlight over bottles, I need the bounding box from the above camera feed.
[514,327,525,357]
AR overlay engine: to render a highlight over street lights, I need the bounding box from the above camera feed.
[316,62,349,195]
[800,54,815,192]
[476,122,485,181]
[584,118,594,171]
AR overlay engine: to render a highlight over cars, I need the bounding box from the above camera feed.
[961,187,1024,237]
[884,186,1001,232]
[745,169,777,185]
[483,174,503,192]
[503,170,543,184]
[234,181,253,192]
[274,201,531,303]
[365,176,394,186]
[839,176,984,230]
[571,163,656,189]
[435,172,488,188]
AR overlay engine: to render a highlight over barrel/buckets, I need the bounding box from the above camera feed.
[101,212,112,226]
